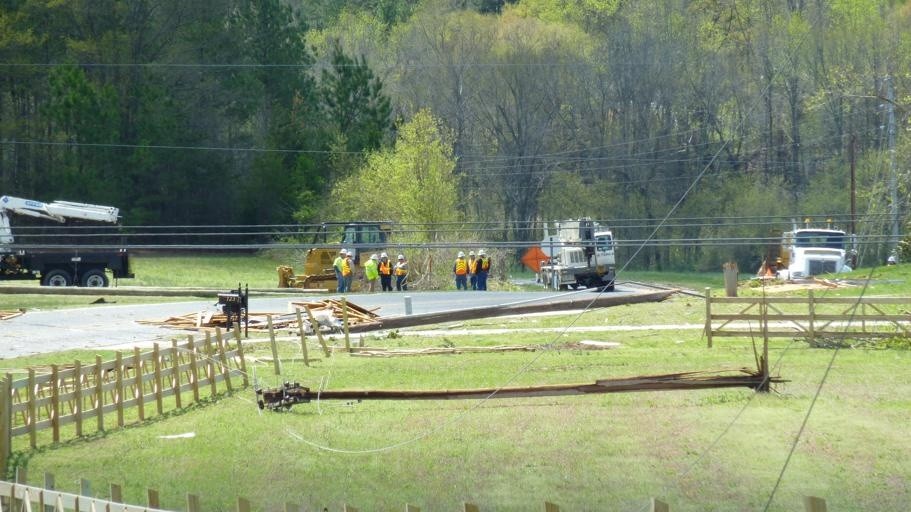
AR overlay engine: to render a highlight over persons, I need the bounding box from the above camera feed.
[341,251,355,292]
[333,248,348,294]
[775,257,786,273]
[364,253,380,292]
[377,253,394,292]
[474,250,491,290]
[467,251,478,290]
[453,252,470,291]
[393,254,408,291]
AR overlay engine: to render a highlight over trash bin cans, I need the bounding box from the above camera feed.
[722,263,737,297]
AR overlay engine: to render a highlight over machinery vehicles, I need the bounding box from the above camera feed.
[277,219,394,292]
[540,216,619,292]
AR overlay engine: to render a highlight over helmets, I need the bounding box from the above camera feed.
[340,249,352,256]
[371,252,403,259]
[458,250,485,257]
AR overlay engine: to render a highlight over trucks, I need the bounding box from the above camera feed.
[776,228,860,282]
[0,210,134,287]
